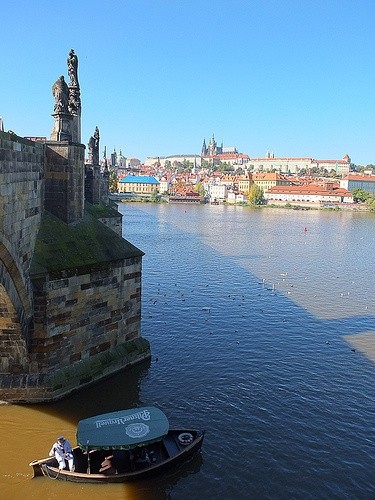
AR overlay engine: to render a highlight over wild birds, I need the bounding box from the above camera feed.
[279,272,288,276]
[258,278,266,284]
[203,303,211,310]
[271,284,275,294]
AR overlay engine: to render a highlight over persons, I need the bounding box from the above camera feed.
[49,436,75,472]
[98,446,157,473]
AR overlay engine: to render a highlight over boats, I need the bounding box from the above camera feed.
[29,406,205,484]
